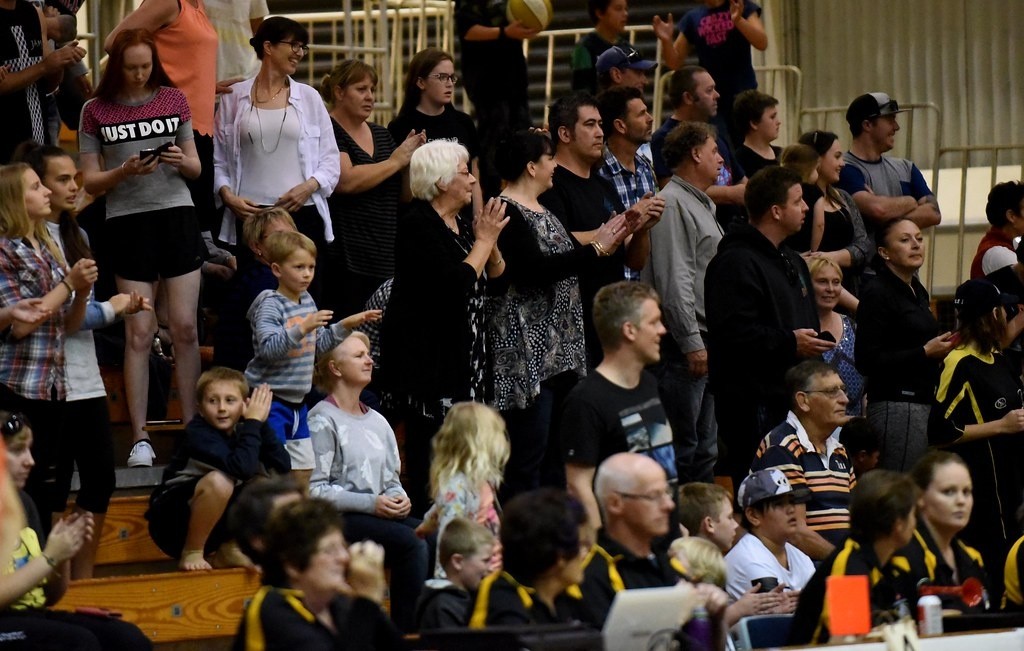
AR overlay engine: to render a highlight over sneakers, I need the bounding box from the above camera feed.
[127,440,155,468]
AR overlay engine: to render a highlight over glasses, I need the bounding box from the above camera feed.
[279,40,310,55]
[615,485,673,502]
[803,386,845,398]
[0,412,25,437]
[425,72,458,85]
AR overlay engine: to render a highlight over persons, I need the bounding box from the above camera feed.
[0,0,1023,651]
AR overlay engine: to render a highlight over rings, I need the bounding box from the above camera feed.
[611,229,616,234]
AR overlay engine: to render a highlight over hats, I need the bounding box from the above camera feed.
[845,92,912,128]
[954,278,1009,318]
[595,46,659,76]
[737,468,808,510]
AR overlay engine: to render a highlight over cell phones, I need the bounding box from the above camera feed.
[140,149,155,166]
[815,331,836,344]
[946,331,962,345]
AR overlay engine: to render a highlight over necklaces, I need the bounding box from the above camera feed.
[254,79,289,153]
[256,77,284,103]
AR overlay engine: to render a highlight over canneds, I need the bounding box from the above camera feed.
[917,595,943,637]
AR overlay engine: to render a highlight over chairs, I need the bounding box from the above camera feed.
[739,614,796,651]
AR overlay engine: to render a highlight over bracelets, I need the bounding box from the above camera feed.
[489,251,503,265]
[122,161,127,178]
[590,240,610,256]
[62,280,74,298]
[41,553,56,568]
